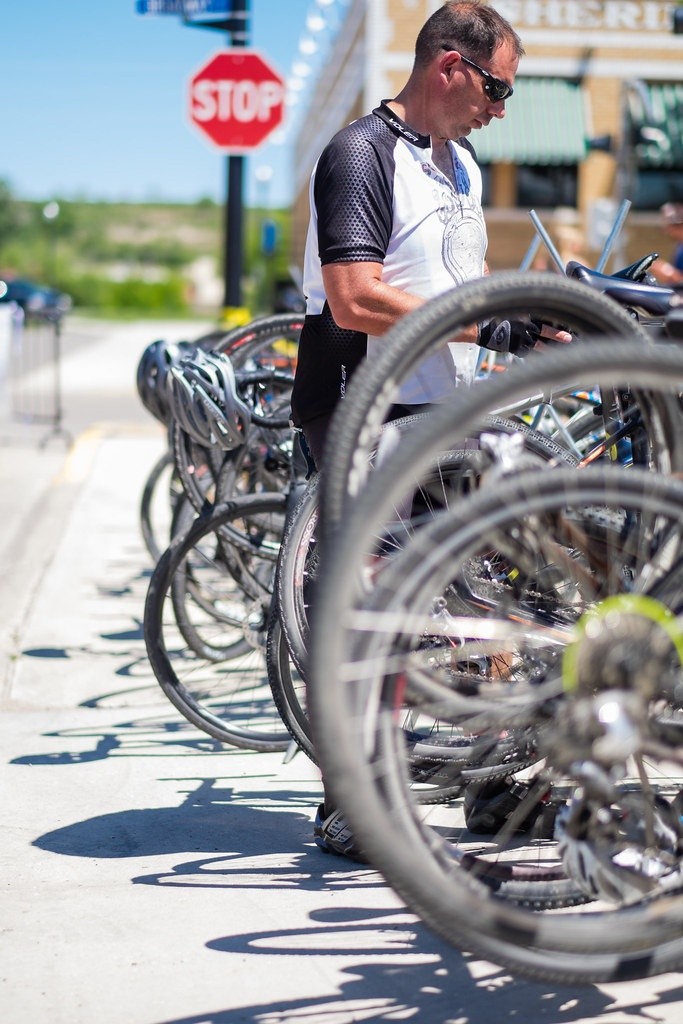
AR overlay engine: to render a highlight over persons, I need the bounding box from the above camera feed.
[661,214,683,294]
[289,0,572,864]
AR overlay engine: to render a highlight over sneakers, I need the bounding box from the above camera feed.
[314,801,369,861]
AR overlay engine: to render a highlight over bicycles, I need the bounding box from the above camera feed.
[140,259,683,977]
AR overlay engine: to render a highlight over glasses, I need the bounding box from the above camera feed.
[442,45,514,103]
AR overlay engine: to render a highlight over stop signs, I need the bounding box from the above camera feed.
[190,52,284,151]
